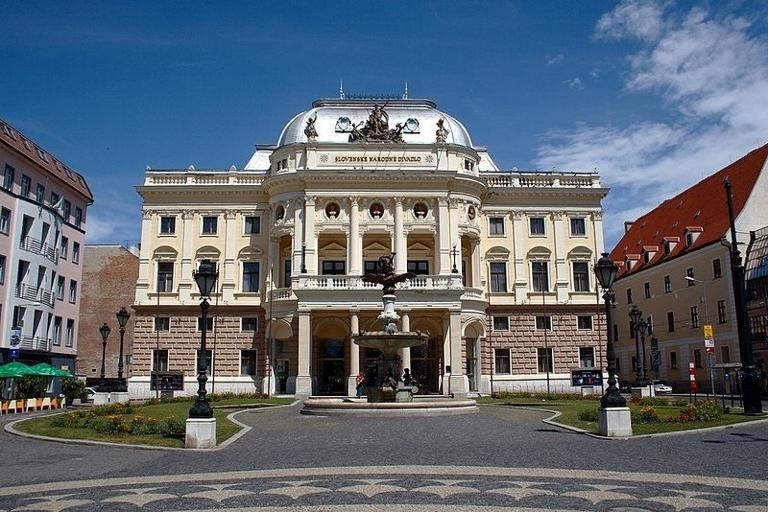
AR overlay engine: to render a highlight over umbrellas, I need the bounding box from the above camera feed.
[1,362,42,380]
[30,361,79,378]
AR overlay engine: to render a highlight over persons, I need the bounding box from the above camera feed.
[351,97,409,143]
[355,370,366,396]
[377,251,395,274]
[303,110,320,138]
[435,117,452,145]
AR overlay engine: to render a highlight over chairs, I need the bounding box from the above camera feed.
[0,397,66,416]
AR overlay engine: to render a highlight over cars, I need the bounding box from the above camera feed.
[649,379,673,394]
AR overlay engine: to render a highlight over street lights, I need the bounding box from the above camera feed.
[116,306,131,383]
[593,252,626,408]
[188,265,218,418]
[638,318,650,385]
[99,323,111,386]
[628,305,646,387]
